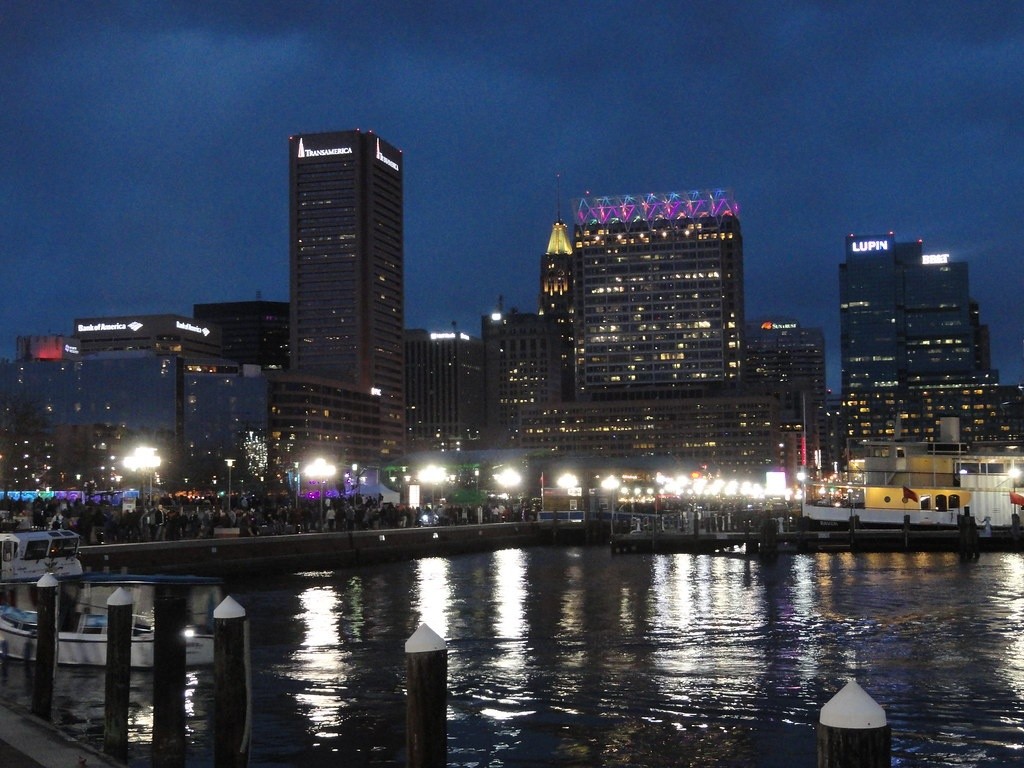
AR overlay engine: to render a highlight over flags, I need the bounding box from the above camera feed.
[904,486,918,502]
[1010,492,1024,506]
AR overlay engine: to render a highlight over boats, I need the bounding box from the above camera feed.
[800,433,1024,531]
[0,528,227,668]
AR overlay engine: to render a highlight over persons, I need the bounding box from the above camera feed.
[0,486,541,547]
[613,488,796,533]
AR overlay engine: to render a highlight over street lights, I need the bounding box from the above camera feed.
[224,459,238,511]
